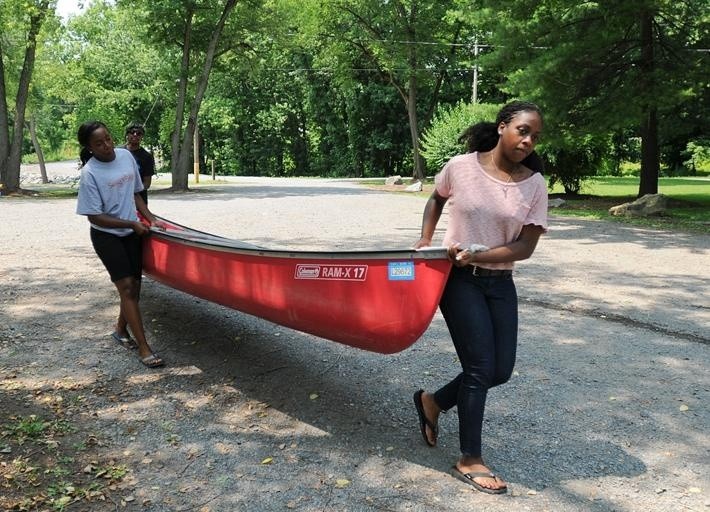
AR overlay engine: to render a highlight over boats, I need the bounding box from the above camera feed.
[135,210,460,355]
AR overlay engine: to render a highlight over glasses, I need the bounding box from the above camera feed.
[128,129,143,136]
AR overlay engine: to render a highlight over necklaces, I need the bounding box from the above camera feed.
[490,150,515,195]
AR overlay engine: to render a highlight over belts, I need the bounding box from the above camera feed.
[464,262,513,278]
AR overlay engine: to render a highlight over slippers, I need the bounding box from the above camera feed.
[414,389,439,447]
[451,459,507,494]
[137,348,164,368]
[113,330,138,349]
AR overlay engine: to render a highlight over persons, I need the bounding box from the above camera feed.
[413,100,550,494]
[116,126,156,210]
[76,119,167,367]
